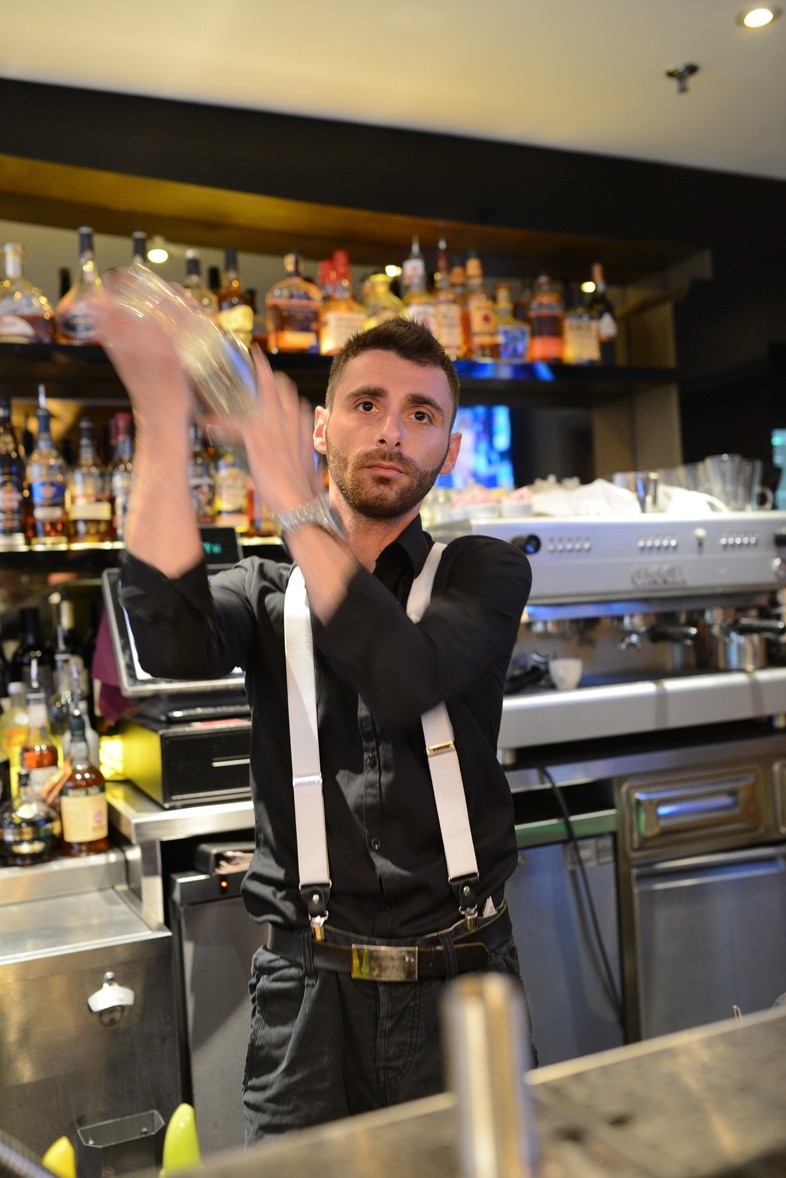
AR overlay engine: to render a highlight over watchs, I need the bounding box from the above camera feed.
[275,496,347,544]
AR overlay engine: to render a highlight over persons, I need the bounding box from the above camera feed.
[83,261,533,1147]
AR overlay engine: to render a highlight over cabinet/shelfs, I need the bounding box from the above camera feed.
[0,335,676,577]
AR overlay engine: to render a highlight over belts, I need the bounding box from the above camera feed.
[268,900,513,981]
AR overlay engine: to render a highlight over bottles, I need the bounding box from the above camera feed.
[0,593,112,867]
[0,405,331,551]
[2,226,622,370]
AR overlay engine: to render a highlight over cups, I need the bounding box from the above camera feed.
[612,454,775,514]
[548,657,582,690]
